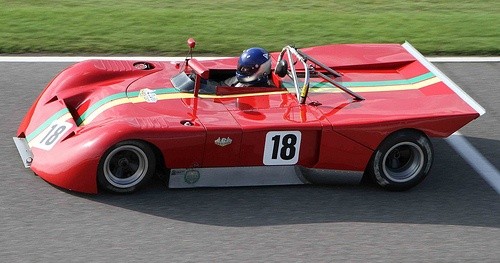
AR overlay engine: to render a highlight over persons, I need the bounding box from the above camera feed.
[188,47,273,88]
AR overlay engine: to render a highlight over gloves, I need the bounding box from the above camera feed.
[189,74,207,88]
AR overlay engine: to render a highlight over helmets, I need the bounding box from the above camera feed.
[236,48,272,83]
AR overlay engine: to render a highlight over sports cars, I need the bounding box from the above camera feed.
[11,40,486,197]
